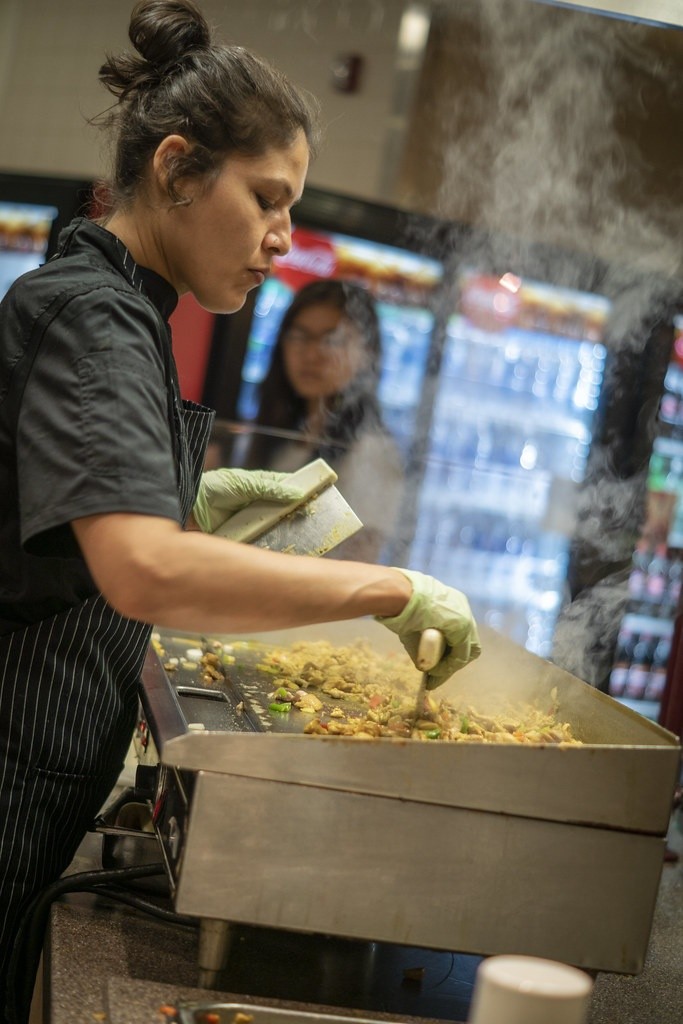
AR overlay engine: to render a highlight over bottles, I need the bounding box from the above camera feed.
[252,298,682,711]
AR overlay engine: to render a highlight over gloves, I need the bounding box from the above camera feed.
[374,567,481,691]
[194,468,302,534]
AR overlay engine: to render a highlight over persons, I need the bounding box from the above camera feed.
[0,0,482,1024]
[244,280,404,564]
